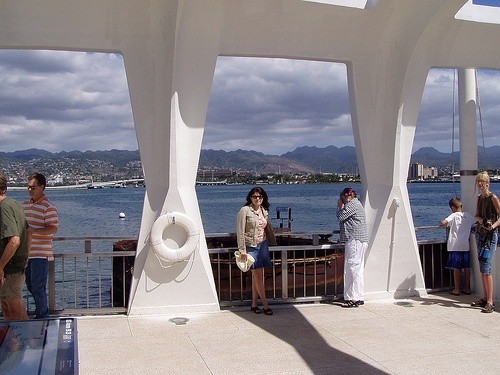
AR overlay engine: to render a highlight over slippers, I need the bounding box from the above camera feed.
[460,289,471,295]
[449,291,459,296]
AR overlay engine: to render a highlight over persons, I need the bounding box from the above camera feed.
[22,173,59,321]
[0,173,30,322]
[440,197,478,295]
[337,187,370,308]
[471,170,500,313]
[235,187,277,315]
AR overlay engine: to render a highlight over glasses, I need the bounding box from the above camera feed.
[26,185,40,191]
[250,195,264,200]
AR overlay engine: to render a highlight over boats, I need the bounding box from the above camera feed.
[113,207,454,306]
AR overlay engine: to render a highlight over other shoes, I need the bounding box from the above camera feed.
[341,299,363,308]
[471,298,487,306]
[483,303,497,313]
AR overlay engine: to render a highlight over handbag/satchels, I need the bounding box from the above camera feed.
[265,210,279,246]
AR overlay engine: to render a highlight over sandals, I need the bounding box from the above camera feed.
[263,307,274,316]
[251,306,262,314]
